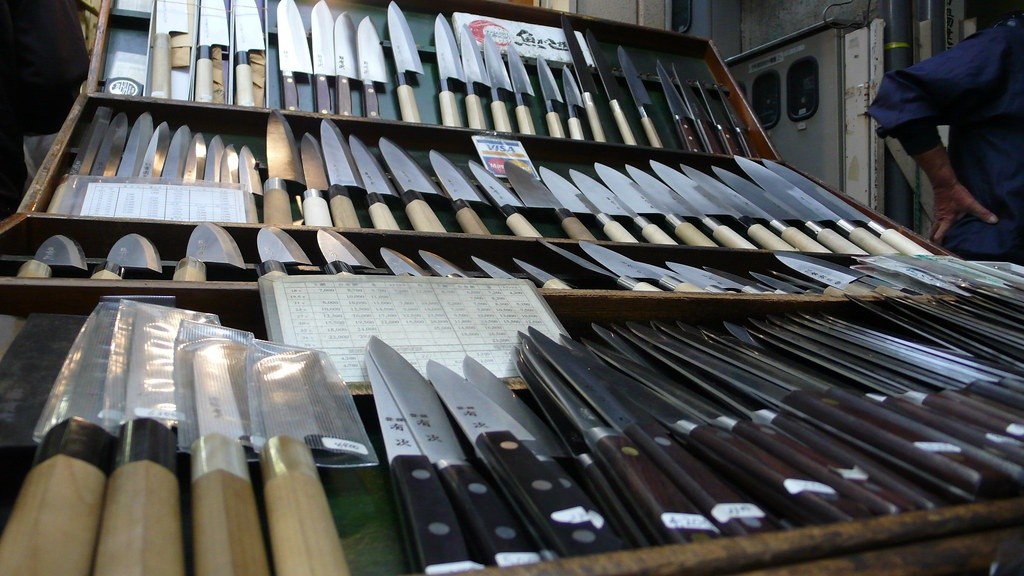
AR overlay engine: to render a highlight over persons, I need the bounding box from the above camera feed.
[867,12,1024,265]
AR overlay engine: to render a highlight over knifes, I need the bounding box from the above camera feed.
[378,136,450,232]
[537,13,638,146]
[468,155,925,296]
[429,148,493,235]
[244,338,379,576]
[717,86,755,158]
[618,45,663,148]
[672,62,724,154]
[364,296,1024,576]
[697,80,740,156]
[67,105,264,224]
[656,58,707,152]
[264,109,306,226]
[150,0,425,123]
[300,117,366,228]
[434,12,536,135]
[349,134,401,230]
[16,223,467,293]
[0,296,269,576]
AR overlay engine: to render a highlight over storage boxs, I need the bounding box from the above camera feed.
[0,0,1024,576]
